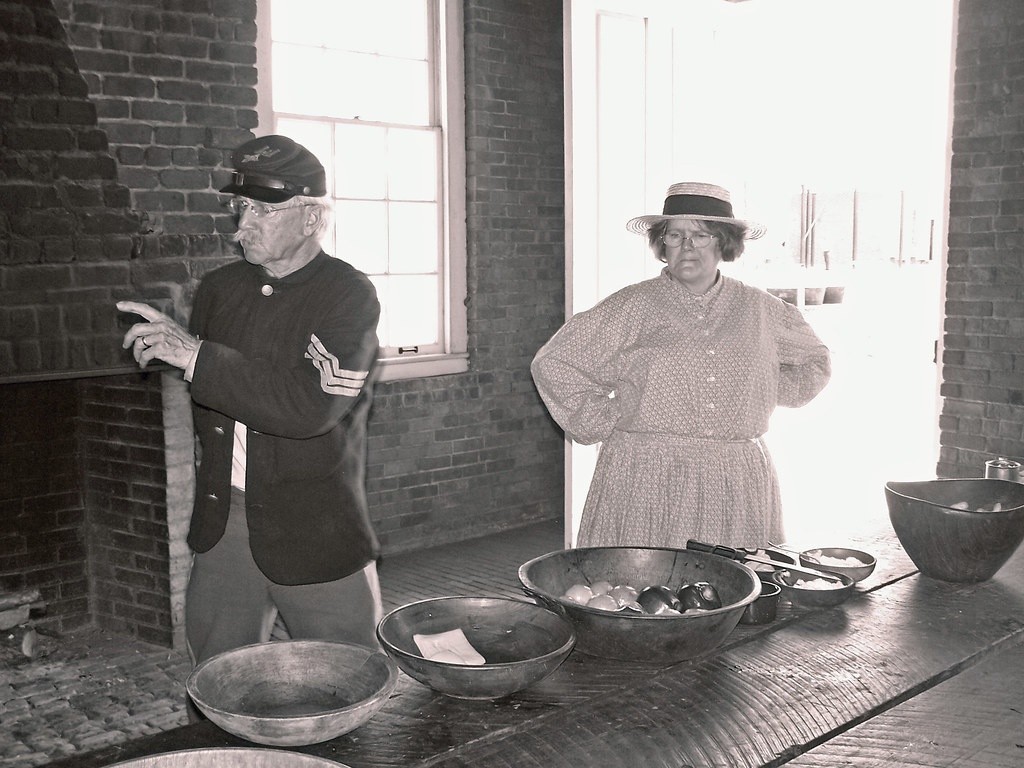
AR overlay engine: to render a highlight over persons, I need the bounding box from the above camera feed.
[116,135,383,670]
[531,183,833,547]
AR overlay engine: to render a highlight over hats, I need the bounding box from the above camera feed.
[218,134,328,203]
[625,182,769,241]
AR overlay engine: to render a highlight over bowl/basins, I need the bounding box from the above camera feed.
[772,570,855,612]
[740,581,782,625]
[186,638,399,748]
[799,548,877,583]
[733,547,796,583]
[99,746,351,768]
[517,545,762,664]
[376,596,578,700]
[883,477,1024,584]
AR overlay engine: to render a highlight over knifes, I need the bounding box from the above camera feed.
[686,538,842,581]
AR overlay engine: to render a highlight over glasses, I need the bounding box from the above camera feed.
[659,231,719,249]
[227,198,319,217]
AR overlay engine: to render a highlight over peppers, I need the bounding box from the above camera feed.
[637,581,722,615]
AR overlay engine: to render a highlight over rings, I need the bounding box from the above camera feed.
[142,337,151,348]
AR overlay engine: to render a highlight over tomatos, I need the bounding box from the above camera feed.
[563,581,643,610]
